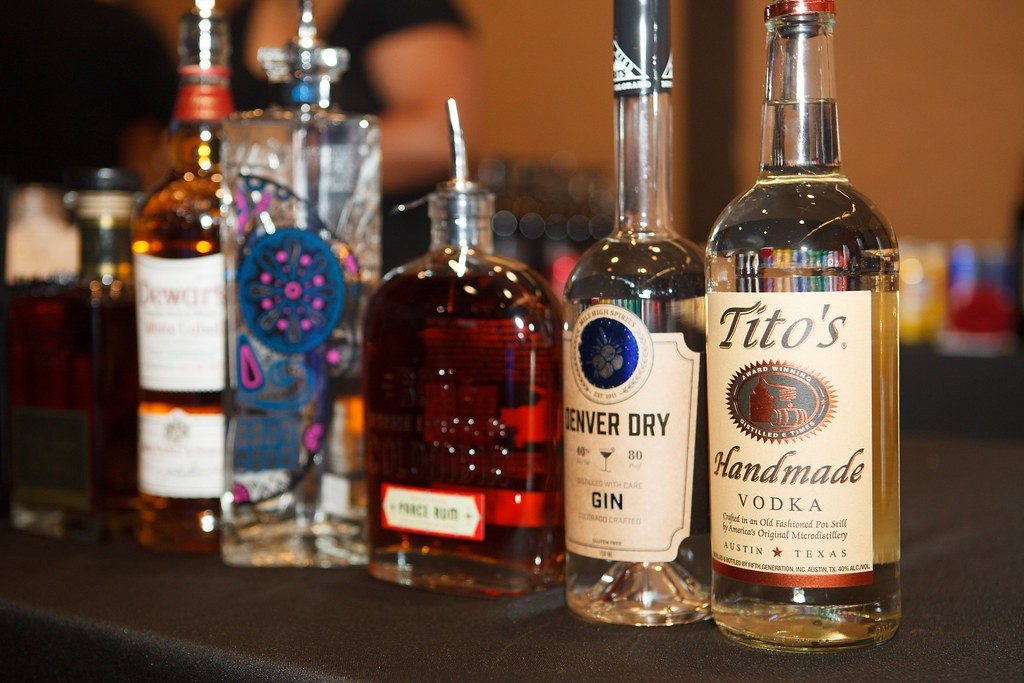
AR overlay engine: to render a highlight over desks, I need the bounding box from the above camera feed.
[2,285,1024,683]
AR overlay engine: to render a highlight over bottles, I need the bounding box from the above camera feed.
[1,0,901,649]
